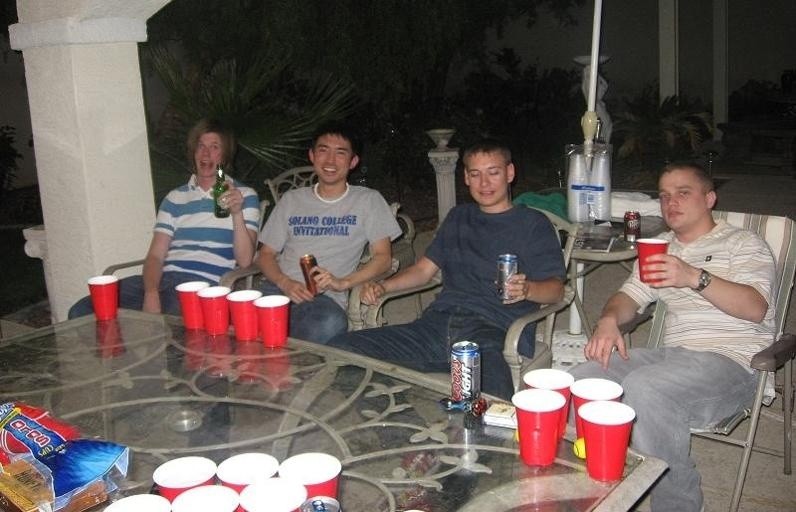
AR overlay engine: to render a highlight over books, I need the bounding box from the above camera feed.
[570,223,624,253]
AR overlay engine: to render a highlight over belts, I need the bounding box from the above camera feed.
[425,302,485,317]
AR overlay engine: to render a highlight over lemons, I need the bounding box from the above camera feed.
[573,437,586,459]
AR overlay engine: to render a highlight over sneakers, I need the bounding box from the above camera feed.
[337,412,429,460]
[398,441,443,478]
[409,434,506,510]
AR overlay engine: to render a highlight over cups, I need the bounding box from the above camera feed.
[569,152,611,223]
[88,276,121,324]
[94,319,126,358]
[636,238,671,285]
[100,451,344,512]
[512,366,636,484]
[172,280,291,349]
[184,330,293,393]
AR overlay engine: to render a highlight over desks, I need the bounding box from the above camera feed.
[529,187,661,367]
[0,301,669,512]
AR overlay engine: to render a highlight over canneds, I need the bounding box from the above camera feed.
[299,253,326,297]
[623,211,641,243]
[450,341,482,401]
[496,254,520,300]
[300,495,342,512]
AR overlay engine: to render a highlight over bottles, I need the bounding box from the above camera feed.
[214,162,230,218]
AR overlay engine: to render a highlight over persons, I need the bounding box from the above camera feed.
[67,116,260,392]
[567,161,777,511]
[175,123,401,348]
[322,135,566,511]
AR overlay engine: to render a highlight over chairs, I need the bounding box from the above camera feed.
[227,205,415,331]
[579,208,794,512]
[363,203,581,395]
[259,163,317,203]
[102,198,265,297]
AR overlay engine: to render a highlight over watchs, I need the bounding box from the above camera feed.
[691,267,712,292]
